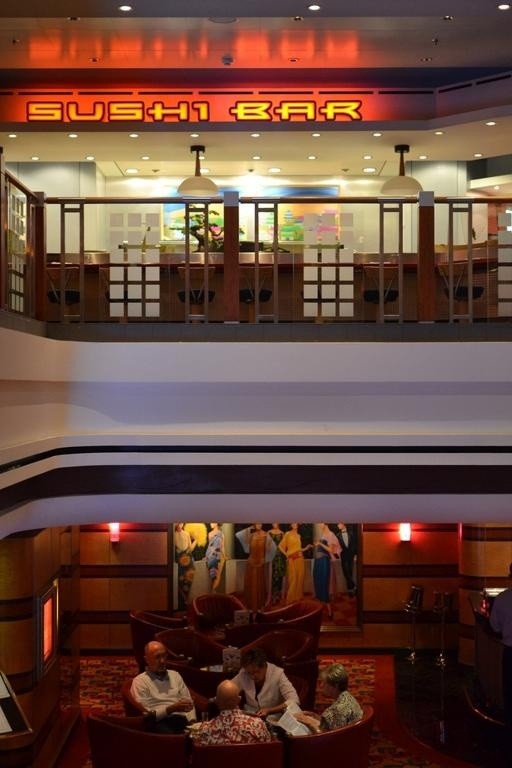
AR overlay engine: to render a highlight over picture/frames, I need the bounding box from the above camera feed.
[165,521,366,635]
[29,572,60,686]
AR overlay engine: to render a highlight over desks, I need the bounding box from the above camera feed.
[351,246,488,323]
[19,252,113,325]
[156,249,306,326]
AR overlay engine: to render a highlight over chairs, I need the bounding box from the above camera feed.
[236,265,274,324]
[41,266,81,324]
[361,264,399,324]
[173,265,217,324]
[436,262,484,323]
[87,707,375,768]
[123,587,323,714]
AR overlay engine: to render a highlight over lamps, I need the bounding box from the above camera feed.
[399,522,412,542]
[178,144,219,197]
[107,522,120,542]
[377,142,426,198]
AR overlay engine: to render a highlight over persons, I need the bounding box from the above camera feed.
[489,565,512,722]
[174,521,356,624]
[192,679,271,746]
[207,651,300,716]
[292,663,364,734]
[129,640,197,732]
